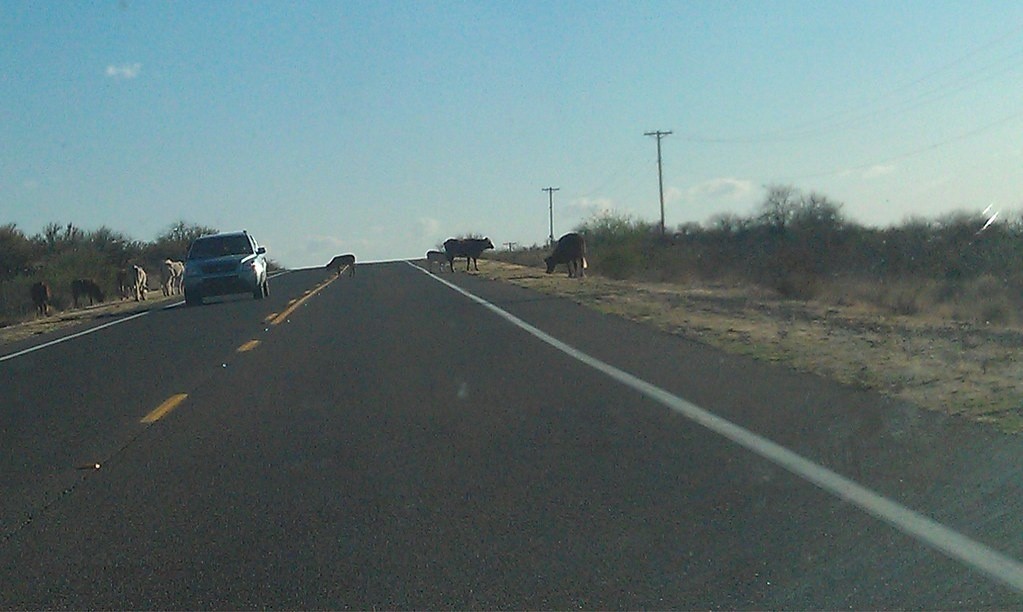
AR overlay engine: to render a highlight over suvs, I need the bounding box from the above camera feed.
[183,231,269,305]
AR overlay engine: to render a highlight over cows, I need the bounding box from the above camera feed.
[160,257,186,298]
[71,278,105,308]
[30,278,53,319]
[426,235,495,274]
[116,265,149,301]
[324,255,356,278]
[543,233,589,278]
